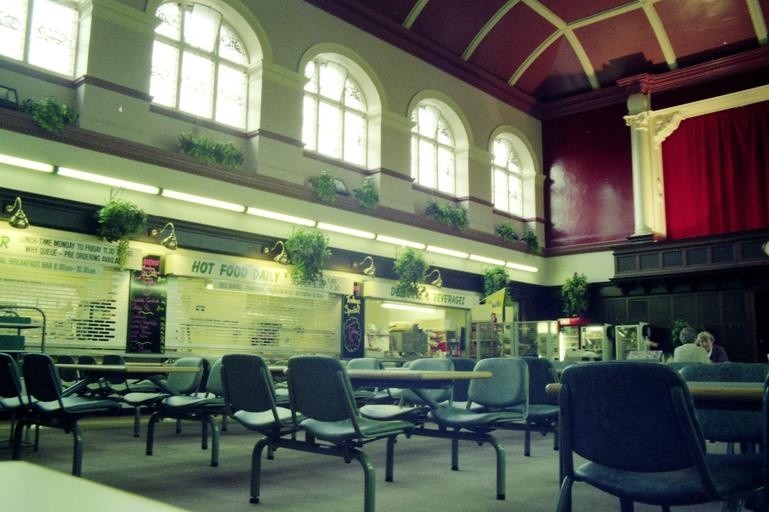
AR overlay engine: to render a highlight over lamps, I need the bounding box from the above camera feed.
[351,255,376,277]
[259,239,290,265]
[149,221,178,251]
[423,268,444,290]
[4,195,30,231]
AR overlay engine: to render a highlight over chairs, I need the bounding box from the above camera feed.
[0,347,768,511]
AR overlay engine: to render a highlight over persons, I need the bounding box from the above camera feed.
[695,330,729,362]
[673,327,712,364]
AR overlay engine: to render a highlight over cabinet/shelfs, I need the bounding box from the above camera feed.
[389,319,650,363]
